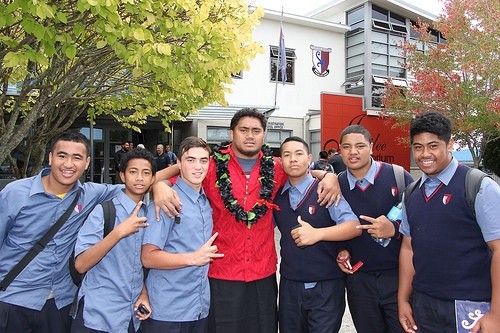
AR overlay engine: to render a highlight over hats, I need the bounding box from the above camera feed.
[137,144,145,149]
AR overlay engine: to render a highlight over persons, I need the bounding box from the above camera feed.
[72,146,155,333]
[113,140,179,185]
[267,137,362,333]
[320,124,420,333]
[327,148,347,175]
[309,150,335,174]
[152,107,345,333]
[141,136,225,333]
[0,132,181,333]
[391,113,500,333]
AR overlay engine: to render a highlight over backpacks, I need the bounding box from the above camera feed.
[68,200,151,317]
[316,160,332,172]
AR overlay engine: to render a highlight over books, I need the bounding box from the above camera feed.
[454,298,492,333]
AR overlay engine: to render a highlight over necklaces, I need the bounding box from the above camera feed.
[212,141,275,226]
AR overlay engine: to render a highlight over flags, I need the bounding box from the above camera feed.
[279,24,288,84]
